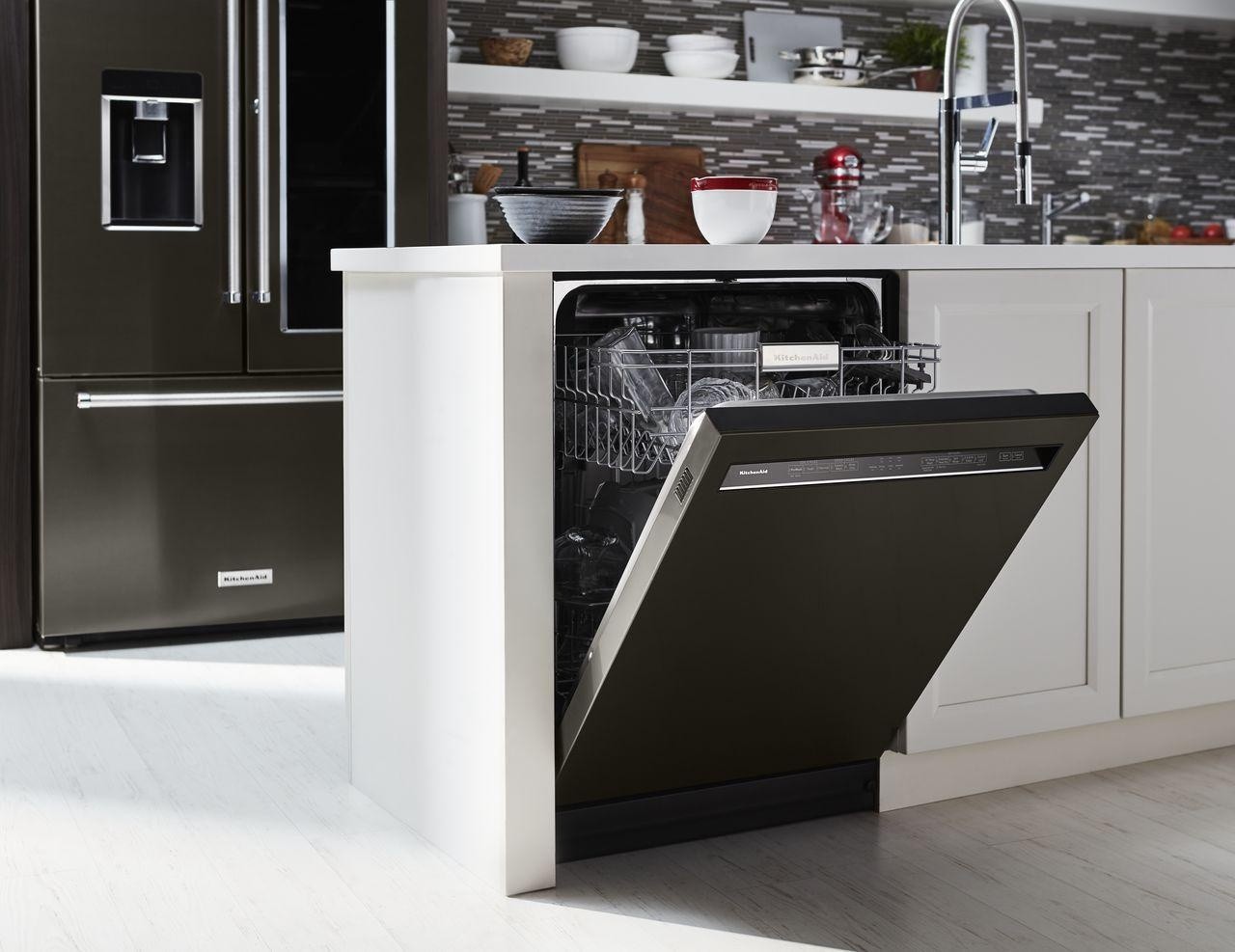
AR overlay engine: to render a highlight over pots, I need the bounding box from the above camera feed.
[777,46,882,68]
[793,66,934,87]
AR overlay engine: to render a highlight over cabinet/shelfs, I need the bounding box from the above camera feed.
[891,269,1235,755]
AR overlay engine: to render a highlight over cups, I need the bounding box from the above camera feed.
[689,176,778,245]
[448,192,489,246]
[800,187,895,245]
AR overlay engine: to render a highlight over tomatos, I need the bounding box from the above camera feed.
[1204,224,1224,238]
[1170,224,1193,239]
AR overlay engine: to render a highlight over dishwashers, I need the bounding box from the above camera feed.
[550,274,1099,867]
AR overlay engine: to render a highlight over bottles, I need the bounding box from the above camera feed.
[598,168,619,245]
[511,147,532,245]
[812,145,864,245]
[626,169,647,246]
[1102,212,1125,244]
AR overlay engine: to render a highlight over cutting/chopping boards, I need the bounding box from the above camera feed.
[1152,236,1235,246]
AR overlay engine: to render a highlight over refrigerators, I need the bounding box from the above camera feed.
[27,0,453,655]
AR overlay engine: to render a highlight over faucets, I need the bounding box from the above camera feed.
[934,0,1038,247]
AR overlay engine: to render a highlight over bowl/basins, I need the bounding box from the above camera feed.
[554,28,640,73]
[665,34,735,53]
[492,186,625,245]
[478,37,533,67]
[660,50,740,79]
